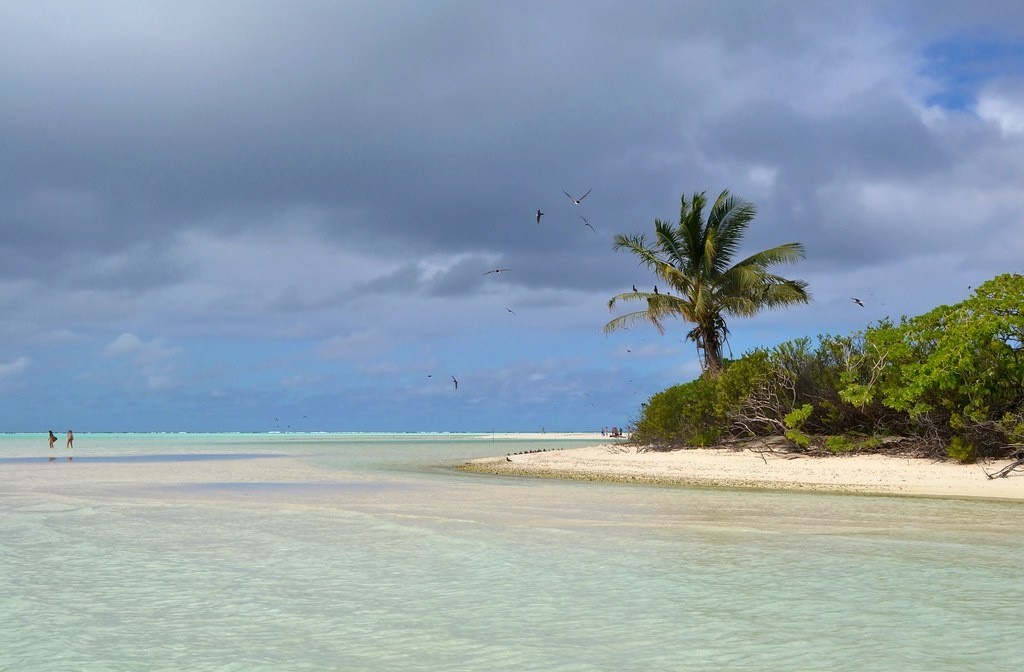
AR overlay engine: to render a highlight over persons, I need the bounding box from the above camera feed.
[48,431,55,448]
[67,430,74,448]
[601,426,623,436]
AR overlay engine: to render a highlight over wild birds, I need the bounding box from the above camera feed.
[428,187,866,410]
